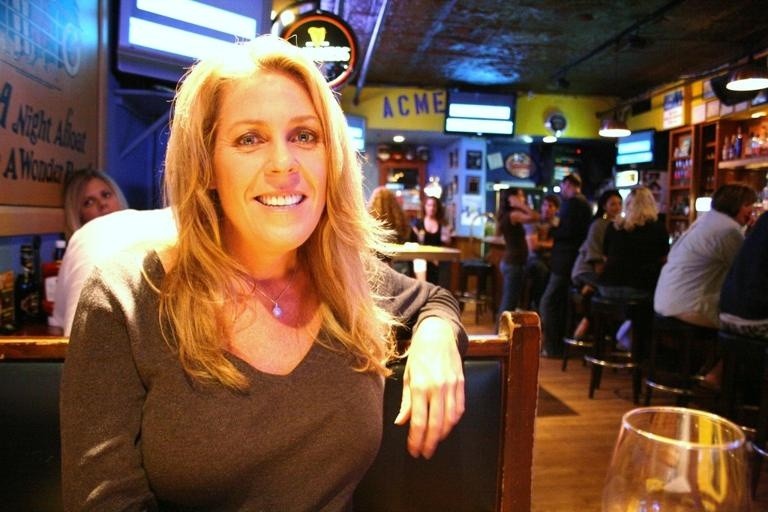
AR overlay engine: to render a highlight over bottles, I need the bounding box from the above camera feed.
[670,132,768,216]
[41,238,67,317]
[13,243,43,327]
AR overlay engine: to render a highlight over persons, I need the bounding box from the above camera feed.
[368,187,450,284]
[63,35,466,511]
[64,169,129,234]
[493,175,768,425]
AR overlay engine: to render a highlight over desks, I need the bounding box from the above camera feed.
[381,243,462,280]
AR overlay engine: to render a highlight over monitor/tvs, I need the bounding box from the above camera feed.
[615,128,655,169]
[116,0,271,85]
[444,88,517,138]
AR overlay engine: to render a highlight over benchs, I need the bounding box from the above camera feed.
[0,310,541,511]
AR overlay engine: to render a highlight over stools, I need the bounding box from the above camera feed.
[459,260,498,325]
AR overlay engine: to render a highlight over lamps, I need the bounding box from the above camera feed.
[726,0,768,92]
[598,40,632,138]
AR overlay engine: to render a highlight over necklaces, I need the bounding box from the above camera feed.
[235,269,301,318]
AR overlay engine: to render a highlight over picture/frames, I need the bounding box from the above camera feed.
[0,0,108,237]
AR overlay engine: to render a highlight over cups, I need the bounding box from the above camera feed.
[601,403,754,512]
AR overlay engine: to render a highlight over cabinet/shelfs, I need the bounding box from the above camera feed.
[666,125,696,242]
